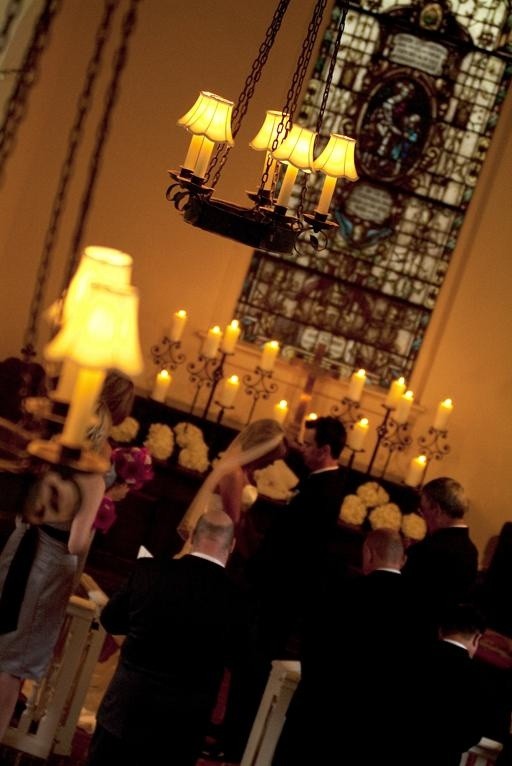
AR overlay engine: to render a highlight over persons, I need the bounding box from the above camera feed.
[1,357,511,765]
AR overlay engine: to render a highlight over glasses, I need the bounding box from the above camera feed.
[416,505,437,515]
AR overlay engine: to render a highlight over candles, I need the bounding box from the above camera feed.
[259,340,280,371]
[344,368,367,404]
[272,399,290,425]
[167,310,188,342]
[434,398,454,430]
[347,418,370,450]
[384,375,414,425]
[217,374,240,408]
[403,456,427,489]
[201,318,242,359]
[298,412,319,445]
[149,369,173,404]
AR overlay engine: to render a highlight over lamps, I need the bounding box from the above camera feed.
[26,245,144,476]
[164,0,362,255]
